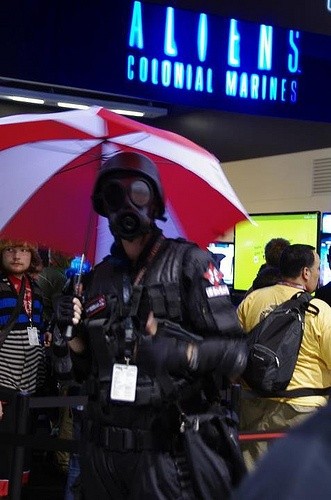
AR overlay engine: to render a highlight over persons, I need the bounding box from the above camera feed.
[236,238,331,474]
[56,152,245,500]
[0,242,73,500]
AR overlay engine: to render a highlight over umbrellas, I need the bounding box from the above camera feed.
[0,107,256,264]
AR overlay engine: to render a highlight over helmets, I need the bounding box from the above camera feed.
[93,151,166,219]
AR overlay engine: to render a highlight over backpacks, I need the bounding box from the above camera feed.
[238,291,330,398]
